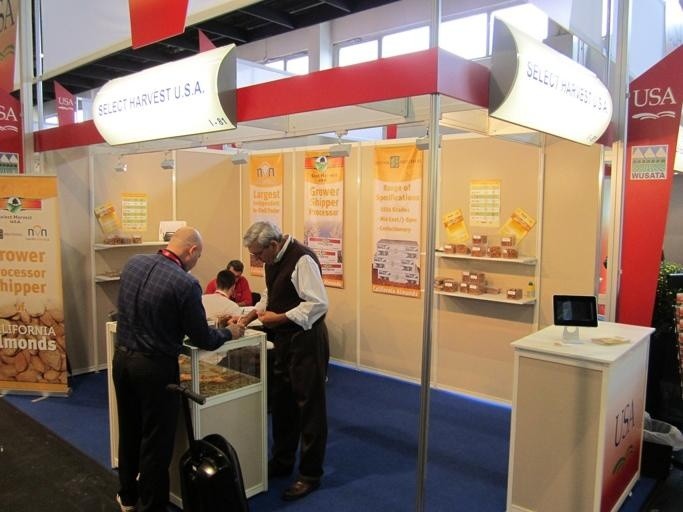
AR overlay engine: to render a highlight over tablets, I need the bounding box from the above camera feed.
[553,294,598,327]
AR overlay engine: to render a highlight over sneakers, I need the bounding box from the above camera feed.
[114,491,137,512]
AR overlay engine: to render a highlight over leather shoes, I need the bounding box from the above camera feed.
[279,477,321,502]
[267,459,290,479]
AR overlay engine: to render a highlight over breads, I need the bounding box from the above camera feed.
[0,298,68,385]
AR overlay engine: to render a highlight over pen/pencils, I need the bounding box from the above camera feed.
[236,309,244,324]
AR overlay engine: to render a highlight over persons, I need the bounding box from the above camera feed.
[226,222,332,502]
[206,261,255,307]
[202,270,243,323]
[112,227,248,512]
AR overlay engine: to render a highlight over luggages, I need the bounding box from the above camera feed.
[162,379,252,512]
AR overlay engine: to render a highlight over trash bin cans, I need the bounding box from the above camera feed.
[641,419,673,481]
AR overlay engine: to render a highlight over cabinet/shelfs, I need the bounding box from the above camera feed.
[93,241,191,283]
[103,319,267,511]
[420,251,536,306]
